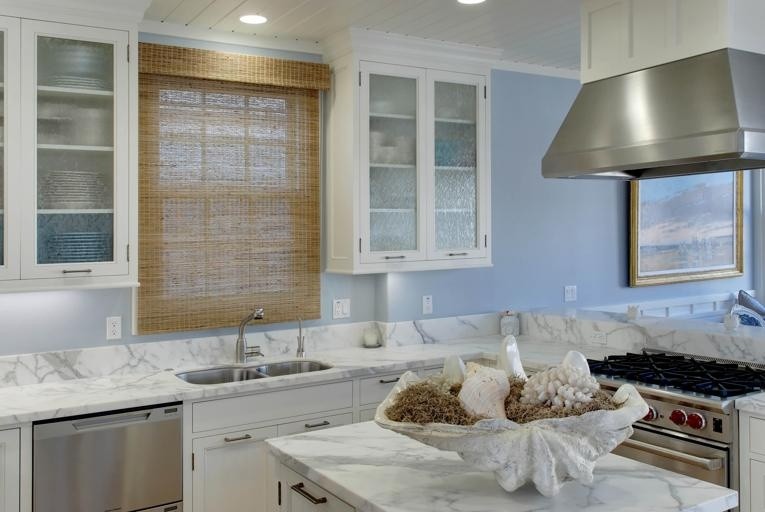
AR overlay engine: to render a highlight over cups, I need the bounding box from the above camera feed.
[369,99,463,167]
[363,328,377,347]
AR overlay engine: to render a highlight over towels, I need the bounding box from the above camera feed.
[587,475,682,512]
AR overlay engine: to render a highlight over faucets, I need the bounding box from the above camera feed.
[235,308,265,364]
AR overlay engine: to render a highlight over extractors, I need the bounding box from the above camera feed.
[539,1,764,177]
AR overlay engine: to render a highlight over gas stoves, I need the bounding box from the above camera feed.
[585,348,765,440]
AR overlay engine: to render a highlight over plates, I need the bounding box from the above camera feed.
[362,343,381,348]
[36,72,113,264]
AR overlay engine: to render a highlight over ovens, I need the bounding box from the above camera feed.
[608,420,739,511]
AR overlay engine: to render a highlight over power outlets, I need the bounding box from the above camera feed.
[106,316,122,340]
[333,298,350,319]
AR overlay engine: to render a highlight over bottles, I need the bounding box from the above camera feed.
[500,315,520,338]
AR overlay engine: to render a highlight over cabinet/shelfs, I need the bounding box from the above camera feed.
[192,380,353,512]
[0,427,20,512]
[319,26,493,276]
[359,371,417,423]
[0,15,140,294]
[750,416,765,512]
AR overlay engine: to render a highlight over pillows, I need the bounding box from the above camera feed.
[739,290,765,316]
[730,303,765,326]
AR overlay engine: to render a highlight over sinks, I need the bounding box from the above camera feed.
[174,366,266,403]
[255,359,334,385]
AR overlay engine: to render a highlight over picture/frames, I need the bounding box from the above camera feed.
[630,170,744,288]
